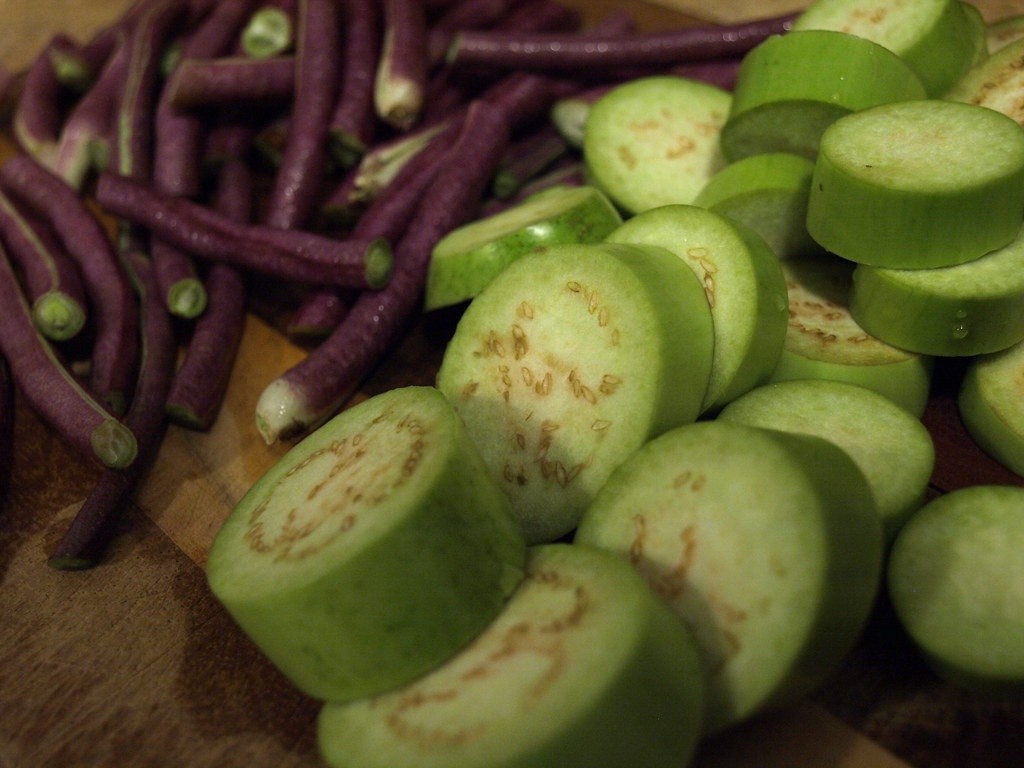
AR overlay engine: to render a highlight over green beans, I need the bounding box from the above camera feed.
[0,0,806,571]
